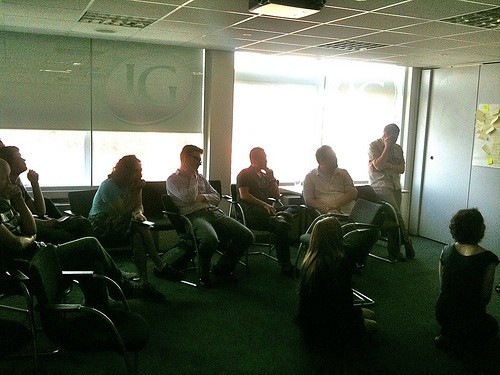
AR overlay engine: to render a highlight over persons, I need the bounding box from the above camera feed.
[293,217,378,331]
[165,145,255,288]
[88,154,186,303]
[0,140,152,314]
[367,123,415,258]
[304,145,381,272]
[430,209,500,347]
[236,147,300,274]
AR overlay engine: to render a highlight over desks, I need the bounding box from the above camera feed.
[278,184,412,198]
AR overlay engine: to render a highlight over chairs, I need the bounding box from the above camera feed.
[0,185,402,375]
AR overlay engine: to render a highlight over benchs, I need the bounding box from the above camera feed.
[142,180,222,230]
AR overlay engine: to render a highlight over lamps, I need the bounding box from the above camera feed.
[248,0,326,18]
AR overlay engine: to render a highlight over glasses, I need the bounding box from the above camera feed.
[190,155,201,162]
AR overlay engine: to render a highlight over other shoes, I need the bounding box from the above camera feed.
[138,283,165,299]
[269,216,292,231]
[405,238,415,259]
[282,265,300,277]
[197,277,208,289]
[109,288,138,301]
[154,263,184,281]
[120,269,137,279]
[212,266,237,282]
[388,253,408,261]
[434,335,451,348]
